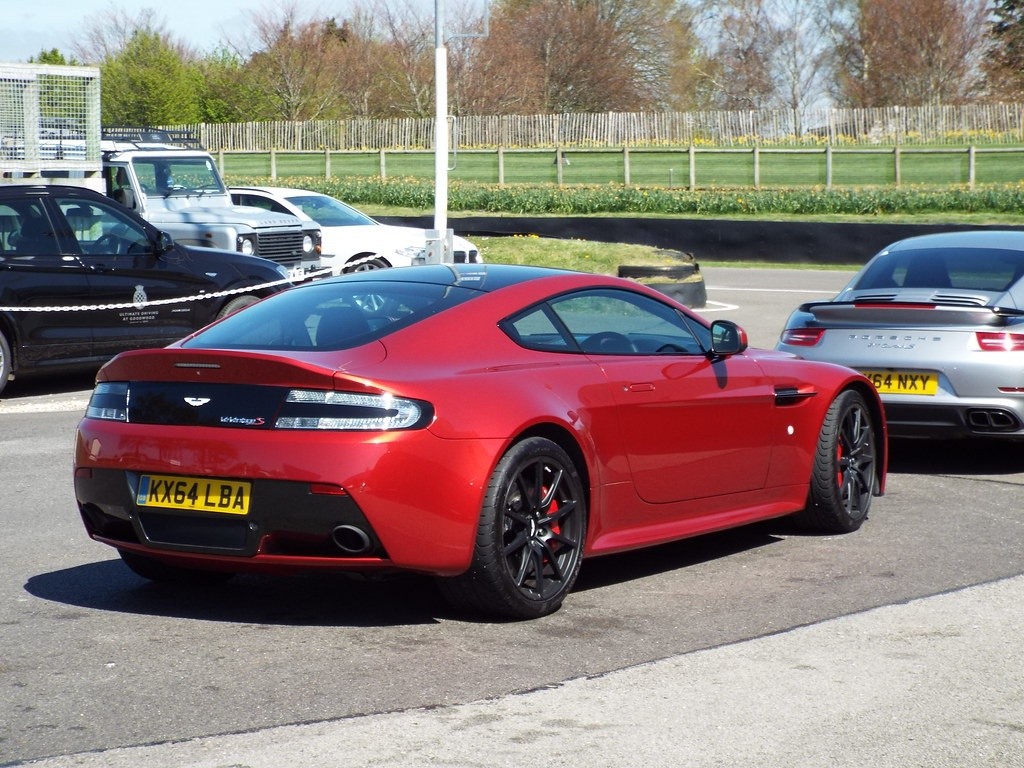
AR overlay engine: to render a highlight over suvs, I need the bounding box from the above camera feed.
[0,124,322,269]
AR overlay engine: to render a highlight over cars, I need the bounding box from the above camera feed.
[0,183,295,398]
[225,185,487,320]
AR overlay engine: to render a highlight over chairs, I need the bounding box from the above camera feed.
[903,252,952,289]
[113,167,136,209]
[16,217,58,257]
[315,306,370,346]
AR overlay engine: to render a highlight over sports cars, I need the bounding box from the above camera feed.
[74,264,888,616]
[773,230,1024,435]
